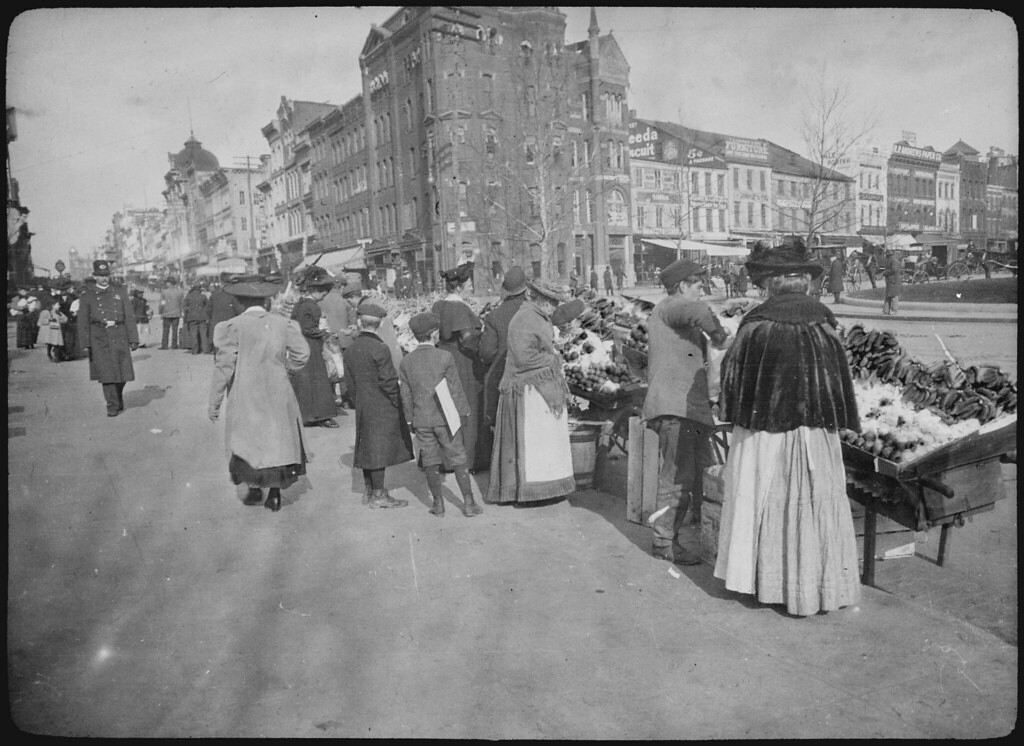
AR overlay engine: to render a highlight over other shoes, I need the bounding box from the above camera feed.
[652,543,700,564]
[320,420,339,429]
[108,411,117,415]
[119,403,123,409]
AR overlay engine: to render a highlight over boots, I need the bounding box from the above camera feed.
[429,496,445,516]
[464,494,483,516]
[362,489,371,505]
[371,489,408,509]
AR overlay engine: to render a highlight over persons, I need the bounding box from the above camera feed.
[913,239,993,279]
[78,260,139,416]
[157,276,246,354]
[133,290,153,347]
[398,314,484,517]
[569,265,627,296]
[11,284,86,363]
[290,265,403,427]
[209,286,311,511]
[809,249,903,314]
[714,247,862,616]
[644,258,725,565]
[433,267,487,473]
[484,278,575,505]
[639,261,662,290]
[479,267,530,432]
[701,262,747,298]
[343,304,414,507]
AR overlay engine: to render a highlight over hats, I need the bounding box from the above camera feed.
[659,258,707,288]
[526,278,571,300]
[293,265,335,285]
[341,283,360,298]
[92,260,111,275]
[408,313,441,337]
[502,268,527,296]
[223,275,279,297]
[356,304,387,318]
[745,236,823,287]
[166,276,176,282]
[551,299,584,324]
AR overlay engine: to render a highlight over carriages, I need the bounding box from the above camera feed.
[718,244,1018,301]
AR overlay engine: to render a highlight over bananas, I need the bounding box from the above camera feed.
[838,325,1017,426]
[580,290,626,340]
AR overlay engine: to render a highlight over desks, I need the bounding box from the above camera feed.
[560,377,647,489]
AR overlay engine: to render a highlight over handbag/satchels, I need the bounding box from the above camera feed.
[50,322,58,328]
[322,336,344,384]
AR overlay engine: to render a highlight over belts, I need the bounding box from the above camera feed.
[90,320,124,327]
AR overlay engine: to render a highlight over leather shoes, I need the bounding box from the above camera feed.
[265,497,281,511]
[244,491,261,504]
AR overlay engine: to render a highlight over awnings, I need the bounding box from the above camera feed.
[639,237,750,257]
[865,234,968,252]
[292,247,364,275]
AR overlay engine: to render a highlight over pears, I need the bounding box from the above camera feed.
[837,428,917,463]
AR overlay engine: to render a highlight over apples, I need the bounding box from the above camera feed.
[563,323,648,391]
